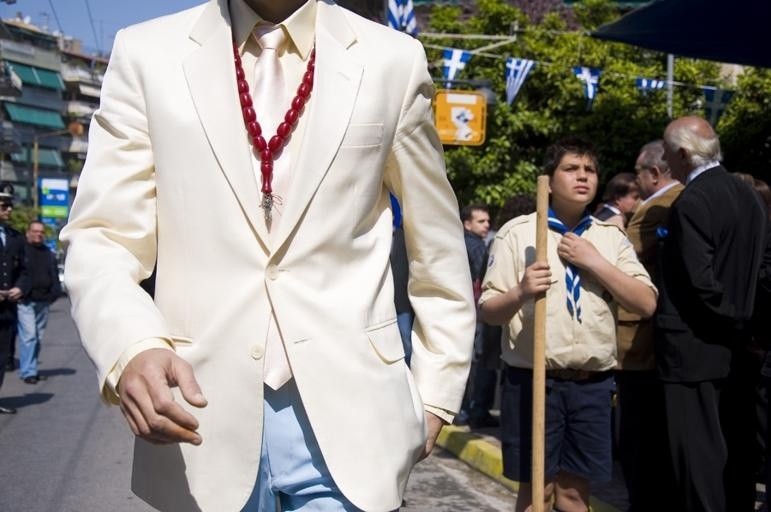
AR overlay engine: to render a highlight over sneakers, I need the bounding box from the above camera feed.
[456,406,500,426]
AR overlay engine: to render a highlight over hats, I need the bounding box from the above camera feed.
[0,183,15,199]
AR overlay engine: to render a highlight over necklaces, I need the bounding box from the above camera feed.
[233,38,315,231]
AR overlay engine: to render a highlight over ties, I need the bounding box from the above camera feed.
[252,24,295,391]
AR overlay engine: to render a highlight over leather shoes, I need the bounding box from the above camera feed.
[26,376,39,384]
[5,363,15,371]
[0,404,16,414]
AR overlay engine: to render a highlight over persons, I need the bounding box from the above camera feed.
[0,180,61,414]
[464,136,659,512]
[604,118,770,512]
[62,0,477,511]
